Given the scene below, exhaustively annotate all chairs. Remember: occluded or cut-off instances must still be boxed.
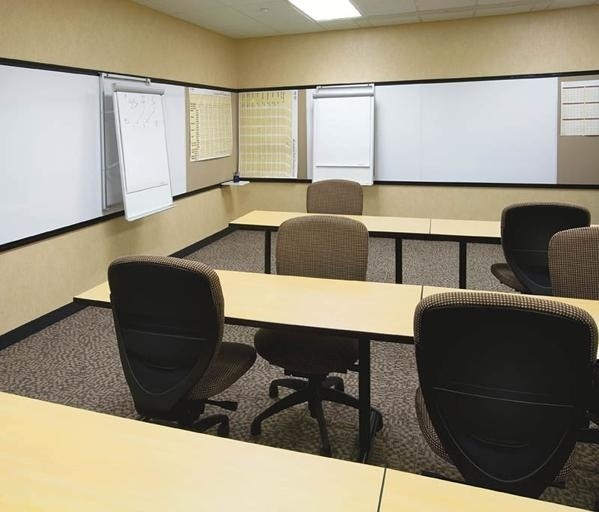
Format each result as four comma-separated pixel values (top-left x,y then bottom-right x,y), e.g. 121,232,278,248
105,181,599,500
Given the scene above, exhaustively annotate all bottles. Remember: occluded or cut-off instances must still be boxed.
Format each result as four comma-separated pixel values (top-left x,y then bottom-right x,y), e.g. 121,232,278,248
233,172,239,182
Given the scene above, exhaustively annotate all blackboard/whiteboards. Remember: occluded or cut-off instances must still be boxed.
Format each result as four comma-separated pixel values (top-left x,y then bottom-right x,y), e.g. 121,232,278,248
312,90,374,187
111,83,175,222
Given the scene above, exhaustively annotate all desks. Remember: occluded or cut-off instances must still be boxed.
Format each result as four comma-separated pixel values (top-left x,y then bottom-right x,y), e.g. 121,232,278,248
71,270,599,463
0,389,587,511
